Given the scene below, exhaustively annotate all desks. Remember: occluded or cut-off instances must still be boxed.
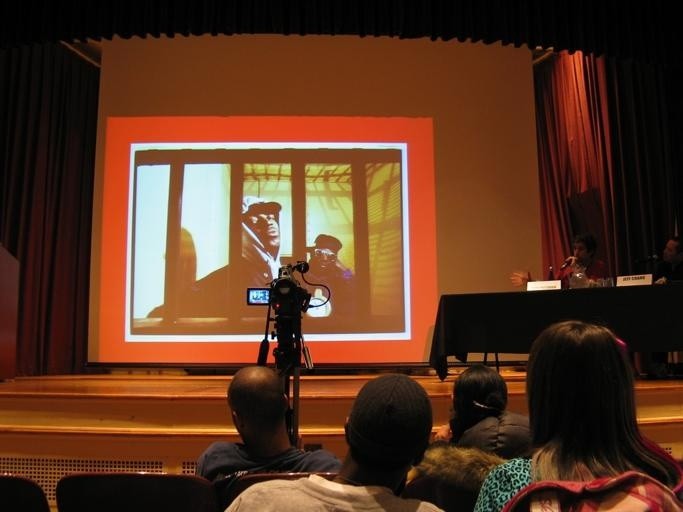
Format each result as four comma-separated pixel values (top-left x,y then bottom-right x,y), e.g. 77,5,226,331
429,281,683,381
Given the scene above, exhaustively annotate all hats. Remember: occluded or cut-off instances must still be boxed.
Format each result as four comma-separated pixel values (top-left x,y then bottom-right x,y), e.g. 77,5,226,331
314,233,343,254
344,371,434,463
241,195,282,219
501,470,683,512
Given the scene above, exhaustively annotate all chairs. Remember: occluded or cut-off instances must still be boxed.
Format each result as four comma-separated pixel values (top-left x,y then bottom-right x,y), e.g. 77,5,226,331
54,473,213,511
2,472,50,511
219,471,345,511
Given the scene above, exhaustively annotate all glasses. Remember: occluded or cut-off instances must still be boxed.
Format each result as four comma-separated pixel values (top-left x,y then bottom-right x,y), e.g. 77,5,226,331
314,248,336,263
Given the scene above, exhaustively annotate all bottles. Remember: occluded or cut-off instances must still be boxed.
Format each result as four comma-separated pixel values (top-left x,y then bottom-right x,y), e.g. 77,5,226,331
548,267,554,280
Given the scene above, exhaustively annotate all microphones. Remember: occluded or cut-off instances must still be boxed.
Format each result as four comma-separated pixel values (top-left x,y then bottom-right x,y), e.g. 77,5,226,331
635,255,658,264
560,258,571,270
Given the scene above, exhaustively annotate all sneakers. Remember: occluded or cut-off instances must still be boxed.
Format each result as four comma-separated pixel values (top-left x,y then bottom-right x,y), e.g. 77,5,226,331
647,363,673,378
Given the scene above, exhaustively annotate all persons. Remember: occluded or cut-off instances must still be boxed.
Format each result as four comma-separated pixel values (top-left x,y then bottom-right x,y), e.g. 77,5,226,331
401,440,506,512
145,194,282,322
642,235,682,380
431,360,536,460
220,374,448,512
472,318,683,512
193,364,342,483
507,232,619,290
305,233,354,317
